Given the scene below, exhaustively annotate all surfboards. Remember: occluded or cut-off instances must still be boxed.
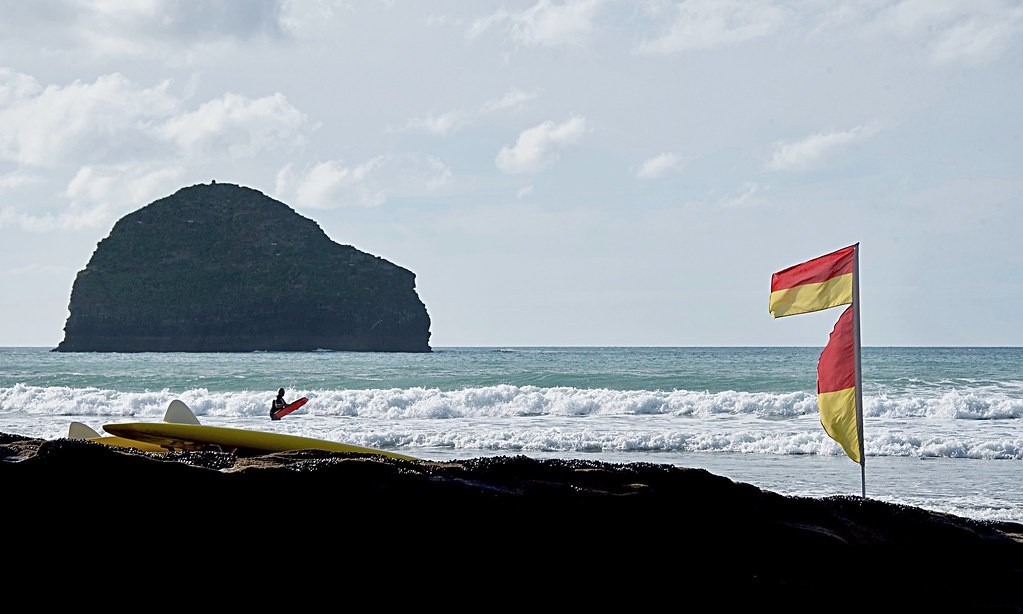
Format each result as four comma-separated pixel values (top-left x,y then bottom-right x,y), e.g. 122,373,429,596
275,397,308,418
67,400,418,461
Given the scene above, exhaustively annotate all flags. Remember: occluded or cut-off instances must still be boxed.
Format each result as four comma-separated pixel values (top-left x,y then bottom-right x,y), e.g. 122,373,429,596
769,244,865,467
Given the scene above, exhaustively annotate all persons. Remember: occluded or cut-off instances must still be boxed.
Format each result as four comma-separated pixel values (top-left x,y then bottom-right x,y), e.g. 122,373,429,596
269,387,289,420
